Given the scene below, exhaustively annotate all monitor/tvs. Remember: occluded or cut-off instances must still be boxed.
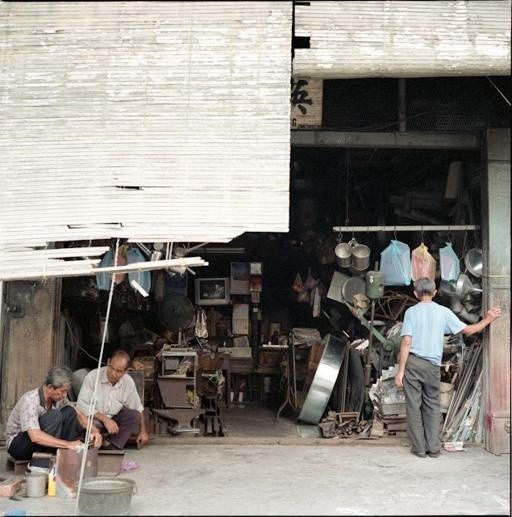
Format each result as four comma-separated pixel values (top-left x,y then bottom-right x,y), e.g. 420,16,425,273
195,277,229,306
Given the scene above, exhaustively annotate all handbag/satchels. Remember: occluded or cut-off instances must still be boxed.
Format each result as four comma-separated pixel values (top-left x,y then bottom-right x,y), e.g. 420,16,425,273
379,239,461,286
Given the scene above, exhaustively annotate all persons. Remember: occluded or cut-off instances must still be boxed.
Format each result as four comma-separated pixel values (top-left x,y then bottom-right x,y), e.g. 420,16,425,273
394,277,501,457
6,367,103,461
77,350,149,450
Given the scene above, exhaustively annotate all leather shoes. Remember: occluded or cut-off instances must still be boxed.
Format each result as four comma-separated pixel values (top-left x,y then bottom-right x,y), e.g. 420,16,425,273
411,448,440,458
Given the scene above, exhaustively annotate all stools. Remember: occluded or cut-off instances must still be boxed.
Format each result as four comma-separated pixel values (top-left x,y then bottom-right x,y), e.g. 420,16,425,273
6,455,30,474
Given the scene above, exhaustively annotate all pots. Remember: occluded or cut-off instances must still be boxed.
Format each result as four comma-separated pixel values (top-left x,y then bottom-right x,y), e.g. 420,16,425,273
70,477,137,515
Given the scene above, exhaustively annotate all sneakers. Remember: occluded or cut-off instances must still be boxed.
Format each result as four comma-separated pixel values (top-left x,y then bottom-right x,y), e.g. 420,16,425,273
99,442,118,450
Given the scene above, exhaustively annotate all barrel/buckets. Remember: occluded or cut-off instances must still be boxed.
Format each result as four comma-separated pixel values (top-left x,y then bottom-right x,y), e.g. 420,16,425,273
75,477,138,517
353,245,370,270
335,243,352,268
